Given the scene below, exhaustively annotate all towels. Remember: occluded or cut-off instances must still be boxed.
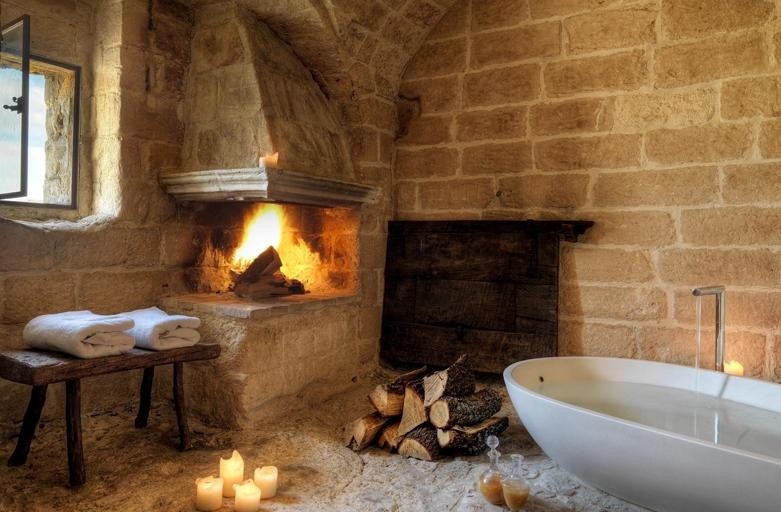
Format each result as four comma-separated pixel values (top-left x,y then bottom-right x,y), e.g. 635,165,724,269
22,305,202,359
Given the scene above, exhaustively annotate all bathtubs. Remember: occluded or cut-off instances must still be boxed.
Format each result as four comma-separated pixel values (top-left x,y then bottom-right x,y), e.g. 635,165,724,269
502,355,781,512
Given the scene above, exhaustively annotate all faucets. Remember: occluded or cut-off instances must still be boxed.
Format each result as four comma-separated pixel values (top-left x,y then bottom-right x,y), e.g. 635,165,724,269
691,285,725,370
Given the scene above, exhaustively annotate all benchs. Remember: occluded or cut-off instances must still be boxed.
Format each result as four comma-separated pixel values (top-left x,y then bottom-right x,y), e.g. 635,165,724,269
2,343,222,486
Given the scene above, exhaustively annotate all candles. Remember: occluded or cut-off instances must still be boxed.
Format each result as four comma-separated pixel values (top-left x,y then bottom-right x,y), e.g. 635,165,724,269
193,449,277,512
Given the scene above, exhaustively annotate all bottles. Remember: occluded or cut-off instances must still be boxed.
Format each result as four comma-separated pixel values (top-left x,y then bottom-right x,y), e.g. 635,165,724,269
478,434,509,506
498,452,533,512
